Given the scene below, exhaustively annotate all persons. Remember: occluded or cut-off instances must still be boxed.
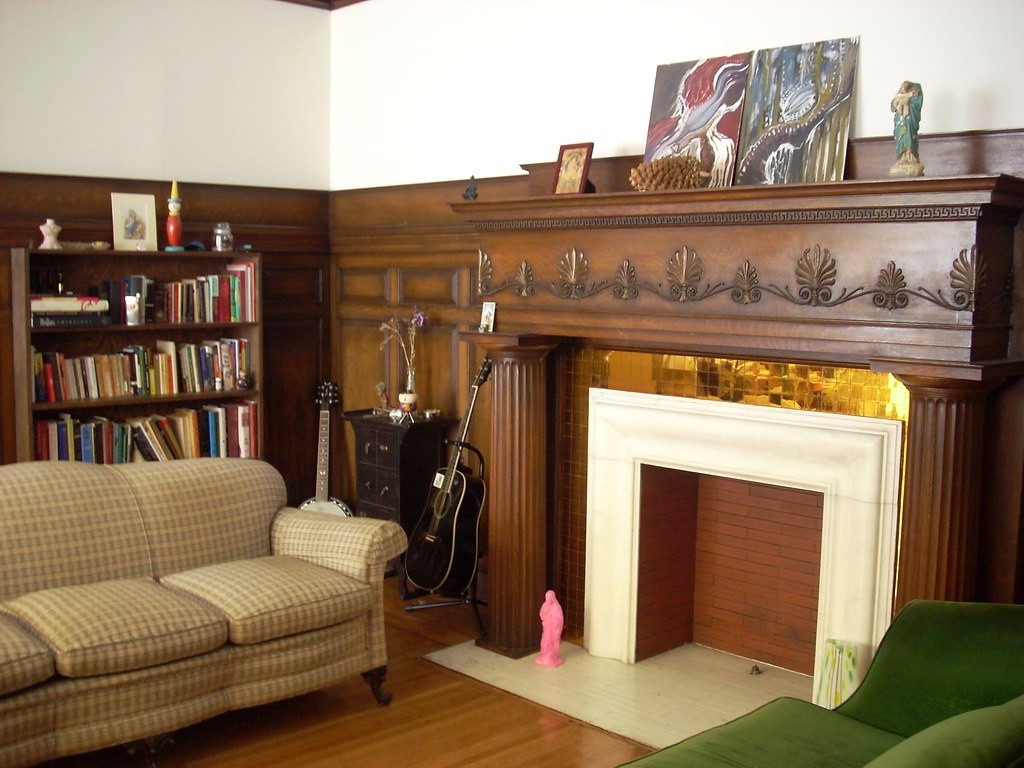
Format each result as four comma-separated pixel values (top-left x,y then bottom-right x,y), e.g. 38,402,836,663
535,590,565,668
462,175,478,200
373,381,392,416
39,219,63,249
891,81,924,163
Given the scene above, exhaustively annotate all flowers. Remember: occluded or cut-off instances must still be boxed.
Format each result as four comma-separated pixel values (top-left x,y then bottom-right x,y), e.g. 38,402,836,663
377,304,432,394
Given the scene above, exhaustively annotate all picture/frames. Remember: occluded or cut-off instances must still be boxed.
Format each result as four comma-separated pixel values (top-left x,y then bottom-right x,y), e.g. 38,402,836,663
480,301,496,333
111,192,158,252
551,142,594,194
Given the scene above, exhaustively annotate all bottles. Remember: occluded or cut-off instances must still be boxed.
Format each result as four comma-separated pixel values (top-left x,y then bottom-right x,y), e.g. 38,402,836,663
211,222,234,252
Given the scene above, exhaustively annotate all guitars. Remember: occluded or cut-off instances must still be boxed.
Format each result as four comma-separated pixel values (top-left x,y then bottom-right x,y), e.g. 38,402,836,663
402,352,492,598
296,379,353,517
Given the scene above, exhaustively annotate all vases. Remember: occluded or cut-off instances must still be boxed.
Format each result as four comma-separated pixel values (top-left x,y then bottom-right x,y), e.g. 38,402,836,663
398,367,421,413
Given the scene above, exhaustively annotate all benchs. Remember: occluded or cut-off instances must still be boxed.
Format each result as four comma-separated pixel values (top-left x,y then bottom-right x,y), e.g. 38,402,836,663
0,457,412,768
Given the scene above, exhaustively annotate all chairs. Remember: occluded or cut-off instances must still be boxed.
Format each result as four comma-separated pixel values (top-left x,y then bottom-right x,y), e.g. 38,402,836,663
611,598,1024,768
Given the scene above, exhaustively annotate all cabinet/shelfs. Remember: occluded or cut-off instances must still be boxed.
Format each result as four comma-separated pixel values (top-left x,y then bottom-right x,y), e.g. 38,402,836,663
10,246,267,465
339,407,461,571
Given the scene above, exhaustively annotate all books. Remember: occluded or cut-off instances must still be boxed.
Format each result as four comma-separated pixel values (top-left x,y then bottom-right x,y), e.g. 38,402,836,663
29,261,258,464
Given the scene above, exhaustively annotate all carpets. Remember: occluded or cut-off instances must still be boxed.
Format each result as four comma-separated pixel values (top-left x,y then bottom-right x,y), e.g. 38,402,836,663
422,636,815,751
